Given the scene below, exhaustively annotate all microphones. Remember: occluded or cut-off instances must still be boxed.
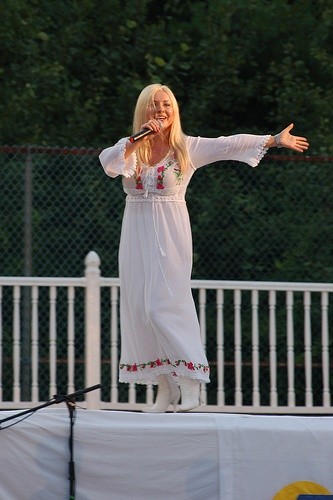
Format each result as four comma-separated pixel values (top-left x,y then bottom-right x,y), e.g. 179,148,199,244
130,120,163,143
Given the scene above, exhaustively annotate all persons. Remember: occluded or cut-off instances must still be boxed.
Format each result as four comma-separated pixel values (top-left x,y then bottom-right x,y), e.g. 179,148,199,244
98,83,309,412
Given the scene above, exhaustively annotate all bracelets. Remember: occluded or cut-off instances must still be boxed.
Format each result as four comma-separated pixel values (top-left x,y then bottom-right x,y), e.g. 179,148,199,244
274,134,282,149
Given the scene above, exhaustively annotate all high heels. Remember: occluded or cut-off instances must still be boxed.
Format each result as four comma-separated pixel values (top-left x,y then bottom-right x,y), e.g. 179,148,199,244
177,382,204,411
143,385,181,413
